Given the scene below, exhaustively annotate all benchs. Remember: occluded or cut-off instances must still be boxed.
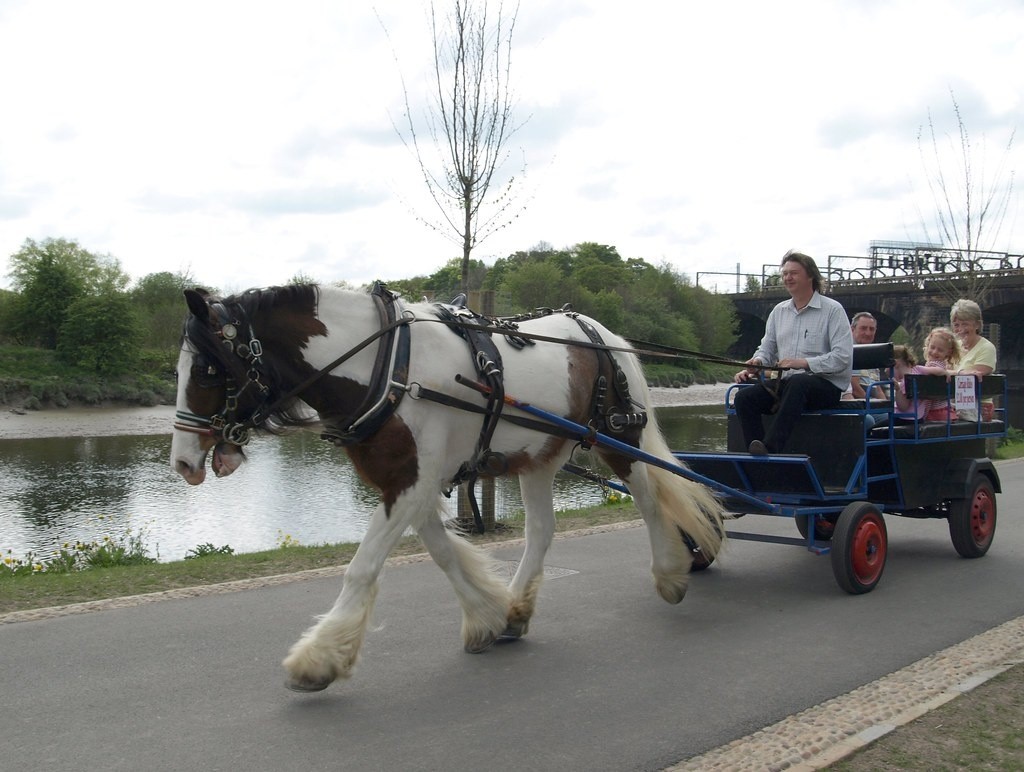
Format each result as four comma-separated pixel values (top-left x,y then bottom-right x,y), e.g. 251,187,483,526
726,341,1010,487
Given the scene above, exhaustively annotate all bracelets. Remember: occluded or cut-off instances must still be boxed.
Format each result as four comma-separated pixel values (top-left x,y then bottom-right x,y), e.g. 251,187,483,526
957,368,965,376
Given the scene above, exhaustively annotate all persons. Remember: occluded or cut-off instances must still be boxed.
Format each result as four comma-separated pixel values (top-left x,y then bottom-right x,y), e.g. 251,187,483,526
923,328,961,421
867,343,951,438
735,360,874,431
851,312,889,430
946,299,996,422
735,253,853,456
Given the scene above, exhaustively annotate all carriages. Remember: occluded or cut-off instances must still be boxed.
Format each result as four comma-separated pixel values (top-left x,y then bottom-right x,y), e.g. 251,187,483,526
169,278,1008,695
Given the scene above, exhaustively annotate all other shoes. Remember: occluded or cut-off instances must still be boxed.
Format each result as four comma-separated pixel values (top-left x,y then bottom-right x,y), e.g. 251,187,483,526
748,440,769,455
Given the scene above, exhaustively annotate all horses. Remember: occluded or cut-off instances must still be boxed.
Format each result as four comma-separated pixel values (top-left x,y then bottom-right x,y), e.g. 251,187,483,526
168,281,736,693
